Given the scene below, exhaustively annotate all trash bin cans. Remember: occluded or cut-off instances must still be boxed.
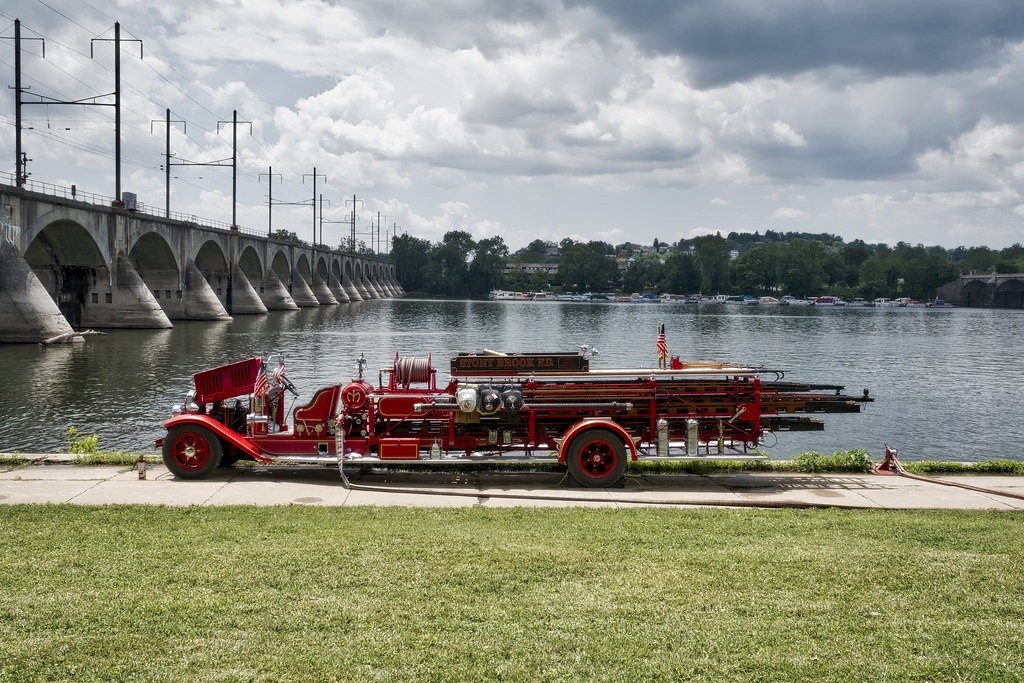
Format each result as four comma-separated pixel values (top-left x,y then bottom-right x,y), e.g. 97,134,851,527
122,192,137,210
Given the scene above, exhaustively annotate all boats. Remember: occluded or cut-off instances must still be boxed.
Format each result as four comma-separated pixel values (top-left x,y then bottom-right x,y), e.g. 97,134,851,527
488,289,954,308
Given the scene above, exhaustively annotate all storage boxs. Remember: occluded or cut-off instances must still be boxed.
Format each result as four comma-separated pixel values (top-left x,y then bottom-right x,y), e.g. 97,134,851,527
378,437,421,460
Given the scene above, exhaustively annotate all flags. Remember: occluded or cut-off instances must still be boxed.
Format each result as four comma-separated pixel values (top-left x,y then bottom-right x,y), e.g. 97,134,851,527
272,357,282,385
254,357,269,400
275,356,286,390
656,323,668,360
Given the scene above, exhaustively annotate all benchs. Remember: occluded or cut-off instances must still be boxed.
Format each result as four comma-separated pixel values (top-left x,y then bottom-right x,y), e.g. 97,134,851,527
292,382,343,438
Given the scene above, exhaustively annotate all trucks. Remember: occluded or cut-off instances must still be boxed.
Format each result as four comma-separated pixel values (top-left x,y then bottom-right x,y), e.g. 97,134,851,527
150,347,762,488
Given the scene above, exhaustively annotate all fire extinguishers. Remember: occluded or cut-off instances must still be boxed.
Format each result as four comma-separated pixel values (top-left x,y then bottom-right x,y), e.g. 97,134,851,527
131,454,147,480
